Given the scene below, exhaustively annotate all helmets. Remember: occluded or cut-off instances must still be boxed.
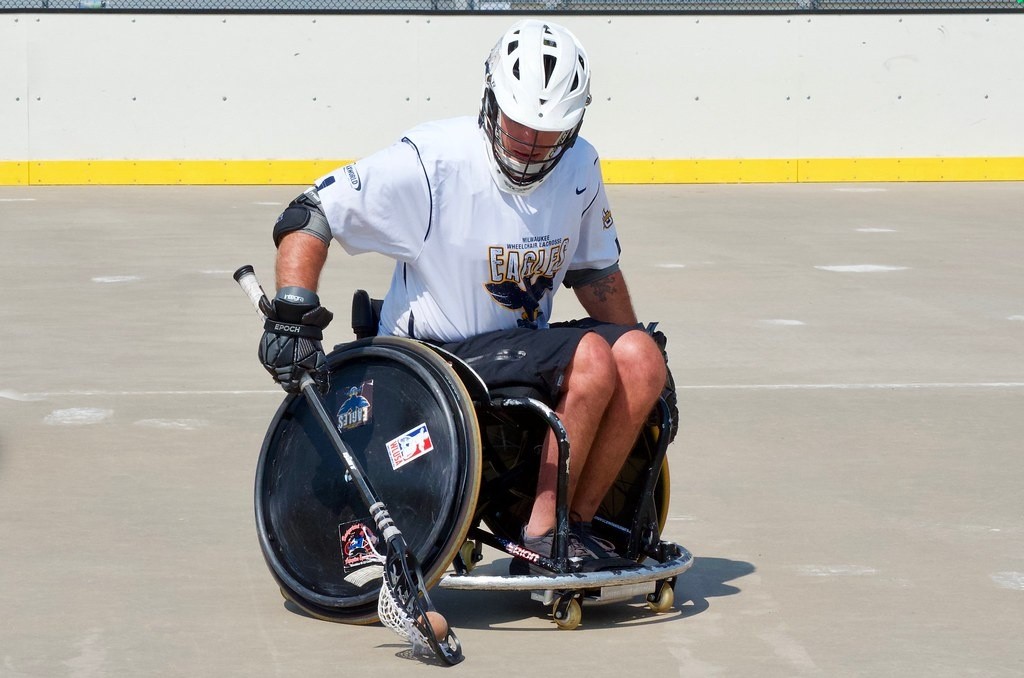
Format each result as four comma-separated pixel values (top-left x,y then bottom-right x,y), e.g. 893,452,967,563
477,19,592,196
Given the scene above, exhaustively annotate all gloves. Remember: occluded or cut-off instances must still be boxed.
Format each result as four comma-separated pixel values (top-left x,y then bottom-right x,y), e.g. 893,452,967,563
256,297,330,398
636,321,680,445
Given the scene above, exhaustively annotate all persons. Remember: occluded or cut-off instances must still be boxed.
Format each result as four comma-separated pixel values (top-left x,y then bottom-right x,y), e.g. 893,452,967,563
257,19,668,573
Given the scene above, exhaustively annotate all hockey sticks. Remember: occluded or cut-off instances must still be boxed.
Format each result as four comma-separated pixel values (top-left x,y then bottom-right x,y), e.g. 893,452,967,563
233,262,463,666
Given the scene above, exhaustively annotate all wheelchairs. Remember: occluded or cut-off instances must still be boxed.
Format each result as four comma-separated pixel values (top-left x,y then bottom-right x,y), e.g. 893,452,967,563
252,290,695,630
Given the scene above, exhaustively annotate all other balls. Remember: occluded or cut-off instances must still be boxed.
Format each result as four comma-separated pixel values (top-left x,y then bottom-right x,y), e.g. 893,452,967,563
415,610,450,642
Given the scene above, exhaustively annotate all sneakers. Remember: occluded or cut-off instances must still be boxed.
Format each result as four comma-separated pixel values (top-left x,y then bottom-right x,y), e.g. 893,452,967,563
522,530,620,566
